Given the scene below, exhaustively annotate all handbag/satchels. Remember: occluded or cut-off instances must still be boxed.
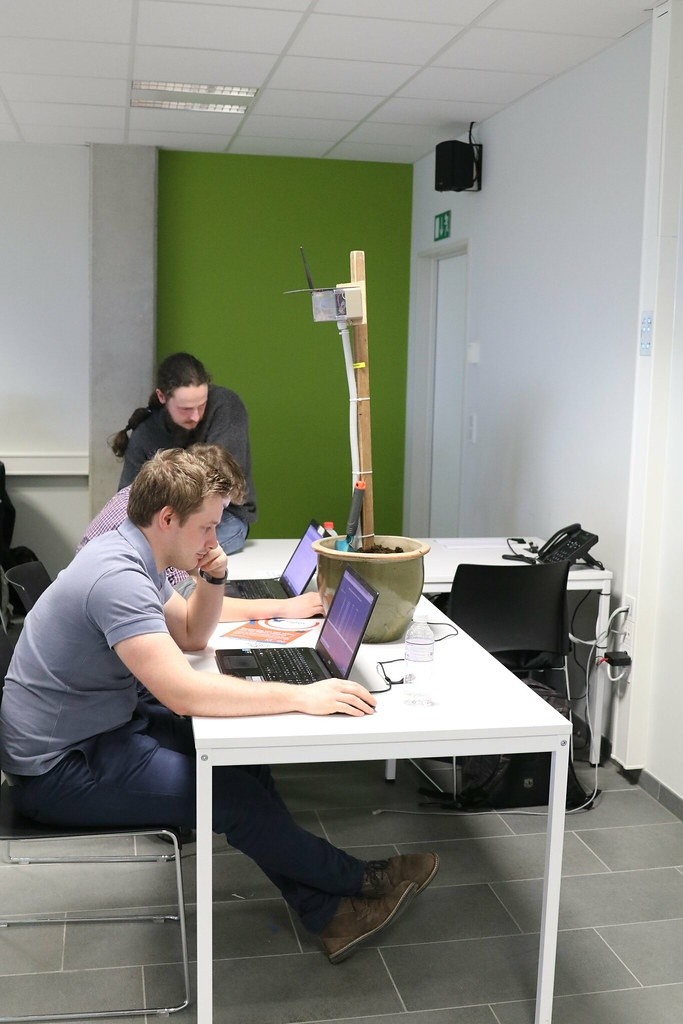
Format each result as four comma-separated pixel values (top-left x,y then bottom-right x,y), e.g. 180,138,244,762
420,752,602,810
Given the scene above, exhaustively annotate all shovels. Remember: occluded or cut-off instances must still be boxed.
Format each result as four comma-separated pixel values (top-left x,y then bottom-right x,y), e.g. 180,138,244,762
335,477,366,553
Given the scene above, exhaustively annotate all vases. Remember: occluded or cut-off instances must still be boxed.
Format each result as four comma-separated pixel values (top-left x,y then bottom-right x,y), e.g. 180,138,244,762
312,535,431,643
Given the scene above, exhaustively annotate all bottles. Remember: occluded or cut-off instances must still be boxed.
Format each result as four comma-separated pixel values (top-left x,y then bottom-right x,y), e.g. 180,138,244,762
323,521,338,536
405,611,435,708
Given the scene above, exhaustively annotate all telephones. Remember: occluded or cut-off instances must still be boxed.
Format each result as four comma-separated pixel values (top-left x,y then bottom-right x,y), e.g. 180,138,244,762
537,523,599,565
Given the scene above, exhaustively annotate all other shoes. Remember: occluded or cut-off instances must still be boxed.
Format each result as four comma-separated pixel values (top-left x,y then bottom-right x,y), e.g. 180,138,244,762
322,880,419,965
362,851,439,894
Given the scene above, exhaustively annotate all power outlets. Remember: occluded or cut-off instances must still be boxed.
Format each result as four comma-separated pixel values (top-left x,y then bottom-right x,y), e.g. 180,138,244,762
620,619,635,649
618,643,634,683
623,594,637,622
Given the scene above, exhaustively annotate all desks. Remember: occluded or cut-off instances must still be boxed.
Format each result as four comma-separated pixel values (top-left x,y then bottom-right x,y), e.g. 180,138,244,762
184,594,573,1024
226,537,613,779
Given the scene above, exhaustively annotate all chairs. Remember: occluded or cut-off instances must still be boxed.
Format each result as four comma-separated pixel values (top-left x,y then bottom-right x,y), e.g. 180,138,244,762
0,559,193,1024
446,559,574,800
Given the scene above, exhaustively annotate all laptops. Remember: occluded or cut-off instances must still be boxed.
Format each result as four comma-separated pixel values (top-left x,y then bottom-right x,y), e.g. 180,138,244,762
215,564,380,686
224,518,332,600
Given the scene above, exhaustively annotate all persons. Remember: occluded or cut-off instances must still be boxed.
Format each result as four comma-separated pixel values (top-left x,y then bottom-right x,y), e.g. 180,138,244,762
0,445,440,964
115,352,257,556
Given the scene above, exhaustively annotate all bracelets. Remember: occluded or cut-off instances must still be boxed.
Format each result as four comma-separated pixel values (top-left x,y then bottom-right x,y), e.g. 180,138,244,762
198,568,228,585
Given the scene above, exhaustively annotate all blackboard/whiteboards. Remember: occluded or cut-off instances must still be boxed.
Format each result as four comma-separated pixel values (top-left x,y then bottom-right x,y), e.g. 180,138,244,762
0,141,92,476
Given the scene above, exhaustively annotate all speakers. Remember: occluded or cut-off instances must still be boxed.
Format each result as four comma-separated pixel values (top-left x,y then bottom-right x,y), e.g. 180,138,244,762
434,140,474,192
